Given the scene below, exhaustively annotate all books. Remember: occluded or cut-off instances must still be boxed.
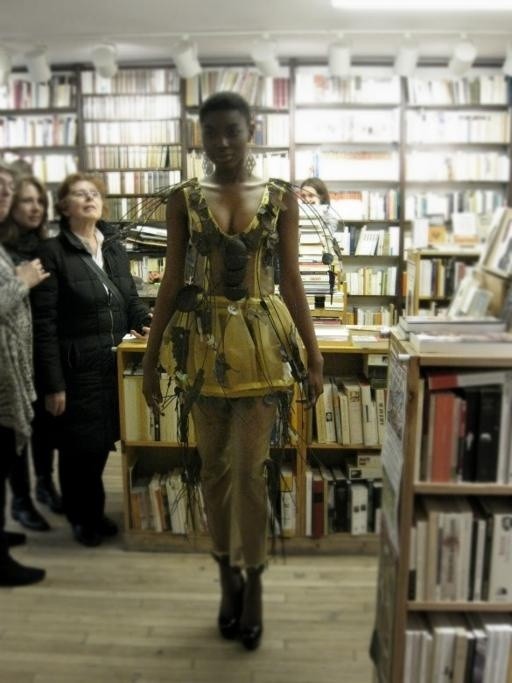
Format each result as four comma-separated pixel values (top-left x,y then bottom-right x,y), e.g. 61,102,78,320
81,68,182,93
126,225,170,247
419,302,451,316
83,95,181,119
409,220,487,247
406,148,510,181
43,224,60,240
409,76,511,104
306,455,382,538
405,187,509,220
409,497,512,603
392,311,512,357
354,334,380,342
103,197,168,220
294,110,401,145
347,304,404,328
84,122,182,144
128,258,167,291
330,225,401,256
406,110,512,144
270,465,297,538
295,74,403,105
1,78,77,108
97,172,181,193
330,189,400,220
0,116,78,147
124,366,196,445
188,149,214,183
85,147,184,170
415,369,512,486
271,381,301,446
43,187,63,220
184,112,205,148
129,463,208,536
186,70,291,109
307,354,391,445
314,325,349,340
404,610,512,682
248,153,291,187
342,266,399,297
449,208,512,310
297,199,346,312
3,154,78,183
296,148,403,182
412,259,471,297
251,112,292,148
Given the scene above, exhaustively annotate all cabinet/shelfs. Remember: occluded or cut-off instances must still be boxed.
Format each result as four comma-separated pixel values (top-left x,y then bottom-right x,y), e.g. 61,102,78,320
116,336,390,555
78,69,185,286
371,332,512,683
0,68,80,241
400,66,512,318
291,63,403,331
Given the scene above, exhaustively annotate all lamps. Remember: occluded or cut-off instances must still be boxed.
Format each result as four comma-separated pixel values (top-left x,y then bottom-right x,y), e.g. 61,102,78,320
394,32,422,79
169,32,203,78
251,33,281,75
448,33,478,75
182,66,292,186
21,46,52,84
328,37,354,74
89,35,120,79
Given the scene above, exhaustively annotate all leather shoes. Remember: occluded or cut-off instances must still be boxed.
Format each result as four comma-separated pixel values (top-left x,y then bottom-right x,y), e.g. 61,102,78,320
1,561,46,585
92,513,120,536
5,529,28,546
74,522,104,546
38,486,64,510
10,498,50,533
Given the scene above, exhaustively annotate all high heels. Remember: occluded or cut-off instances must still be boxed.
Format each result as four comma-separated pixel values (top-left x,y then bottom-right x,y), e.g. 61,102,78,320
240,598,264,647
217,572,245,636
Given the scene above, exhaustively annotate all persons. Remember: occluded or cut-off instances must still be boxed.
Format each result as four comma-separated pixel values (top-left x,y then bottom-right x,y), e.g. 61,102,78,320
38,173,151,547
141,96,324,652
301,179,327,205
0,173,50,268
1,167,52,590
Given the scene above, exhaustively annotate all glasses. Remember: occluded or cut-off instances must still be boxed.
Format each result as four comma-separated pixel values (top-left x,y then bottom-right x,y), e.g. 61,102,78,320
0,179,17,192
65,189,104,199
